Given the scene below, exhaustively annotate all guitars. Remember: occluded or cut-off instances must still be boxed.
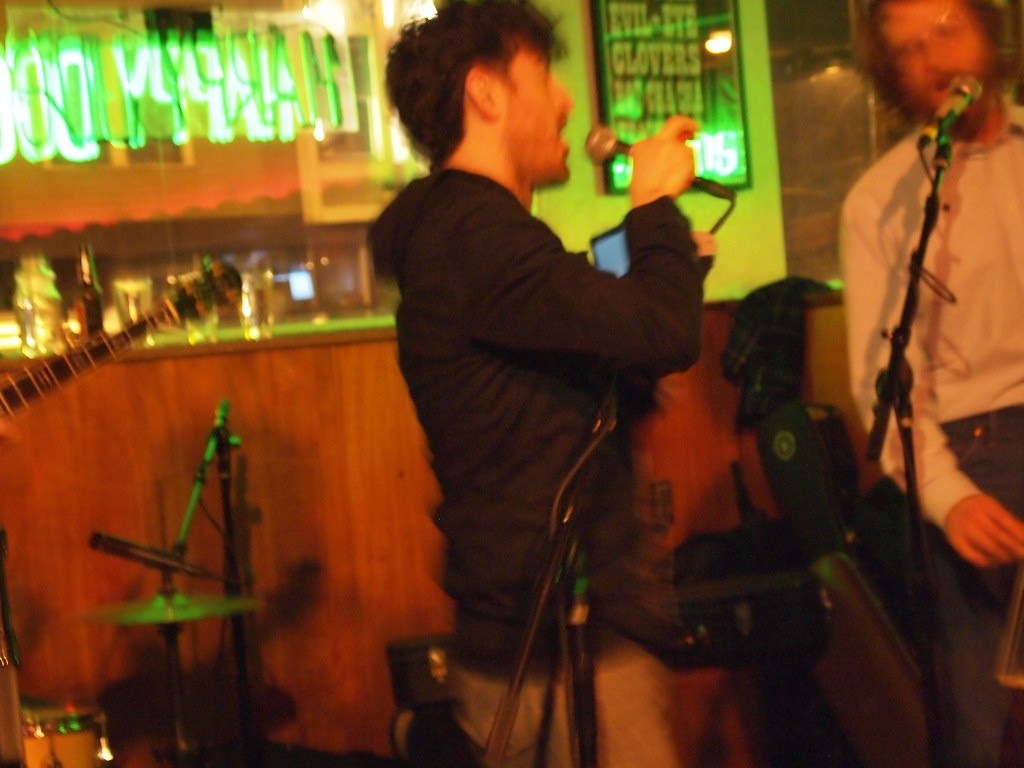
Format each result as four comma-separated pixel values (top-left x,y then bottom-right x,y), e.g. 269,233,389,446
2,259,245,421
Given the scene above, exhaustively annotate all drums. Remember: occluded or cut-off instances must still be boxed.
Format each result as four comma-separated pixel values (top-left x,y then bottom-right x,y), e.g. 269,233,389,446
18,698,115,768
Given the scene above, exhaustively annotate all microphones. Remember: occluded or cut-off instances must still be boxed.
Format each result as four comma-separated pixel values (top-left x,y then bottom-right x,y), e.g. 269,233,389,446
585,125,734,201
89,530,209,578
918,75,982,151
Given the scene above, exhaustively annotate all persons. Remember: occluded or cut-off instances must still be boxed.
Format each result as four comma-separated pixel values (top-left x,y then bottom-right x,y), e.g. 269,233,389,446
837,0,1024,767
362,3,718,768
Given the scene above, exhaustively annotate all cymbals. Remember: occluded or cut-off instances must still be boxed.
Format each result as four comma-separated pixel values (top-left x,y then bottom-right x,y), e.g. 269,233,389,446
78,587,260,626
